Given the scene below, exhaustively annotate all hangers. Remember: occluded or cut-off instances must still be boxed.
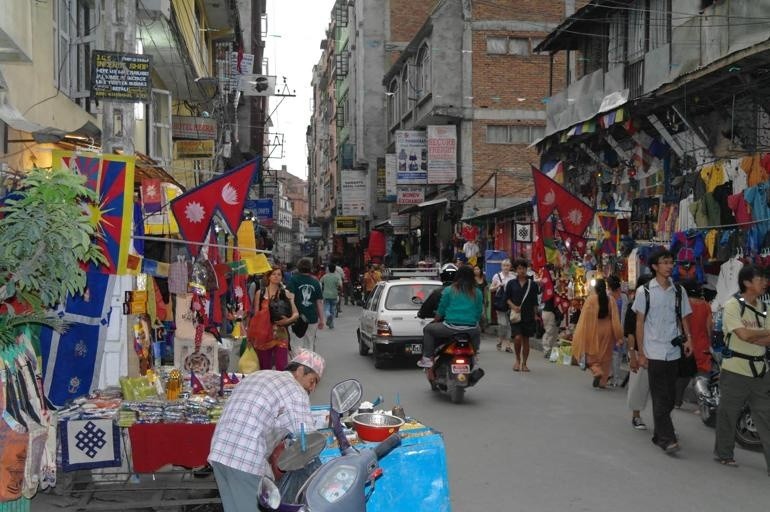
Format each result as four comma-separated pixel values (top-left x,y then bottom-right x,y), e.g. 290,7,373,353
735,247,769,260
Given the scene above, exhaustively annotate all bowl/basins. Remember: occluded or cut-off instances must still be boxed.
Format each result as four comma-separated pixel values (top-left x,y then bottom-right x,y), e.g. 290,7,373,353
351,413,406,443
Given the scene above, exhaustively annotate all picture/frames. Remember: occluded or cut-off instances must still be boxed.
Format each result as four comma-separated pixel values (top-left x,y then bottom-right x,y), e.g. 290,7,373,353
174,339,219,380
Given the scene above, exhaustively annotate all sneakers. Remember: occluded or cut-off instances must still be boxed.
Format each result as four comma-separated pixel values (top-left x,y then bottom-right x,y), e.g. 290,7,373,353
592,374,602,387
631,415,647,431
496,343,502,351
505,346,513,353
544,349,551,358
326,315,333,328
416,357,434,368
652,436,681,453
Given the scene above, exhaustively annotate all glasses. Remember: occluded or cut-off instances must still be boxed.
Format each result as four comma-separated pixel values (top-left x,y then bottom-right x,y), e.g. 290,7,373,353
658,262,674,264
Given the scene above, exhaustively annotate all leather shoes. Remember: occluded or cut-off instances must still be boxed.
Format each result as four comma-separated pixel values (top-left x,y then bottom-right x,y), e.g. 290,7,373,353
513,367,520,371
522,366,530,371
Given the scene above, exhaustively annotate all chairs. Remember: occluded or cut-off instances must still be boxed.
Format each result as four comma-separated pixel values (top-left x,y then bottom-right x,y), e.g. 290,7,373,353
390,292,410,305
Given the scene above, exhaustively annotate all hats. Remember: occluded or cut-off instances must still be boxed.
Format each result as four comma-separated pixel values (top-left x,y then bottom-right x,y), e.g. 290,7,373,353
296,257,312,269
288,350,326,381
439,263,458,275
455,252,467,263
418,261,425,265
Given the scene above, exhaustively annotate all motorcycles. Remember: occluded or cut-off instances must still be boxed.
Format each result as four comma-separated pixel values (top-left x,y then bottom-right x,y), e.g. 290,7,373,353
254,379,403,512
411,297,484,404
693,329,764,451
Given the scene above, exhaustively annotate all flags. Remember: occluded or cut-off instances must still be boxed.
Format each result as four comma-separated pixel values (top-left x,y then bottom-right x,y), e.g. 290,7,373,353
51,148,135,278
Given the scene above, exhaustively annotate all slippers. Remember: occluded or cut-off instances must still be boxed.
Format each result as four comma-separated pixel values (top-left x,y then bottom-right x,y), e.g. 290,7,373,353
715,457,739,466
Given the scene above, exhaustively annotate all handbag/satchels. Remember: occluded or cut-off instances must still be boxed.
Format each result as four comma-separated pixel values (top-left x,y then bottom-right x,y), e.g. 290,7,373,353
491,272,510,313
290,317,308,338
678,349,698,379
269,284,293,328
510,306,522,324
535,319,546,339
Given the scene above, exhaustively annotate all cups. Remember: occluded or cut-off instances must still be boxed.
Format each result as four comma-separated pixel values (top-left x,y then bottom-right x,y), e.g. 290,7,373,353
392,407,405,419
359,408,373,414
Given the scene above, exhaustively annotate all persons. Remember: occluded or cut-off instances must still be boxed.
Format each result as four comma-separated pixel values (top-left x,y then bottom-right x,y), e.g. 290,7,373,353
629,249,694,459
417,254,488,368
572,274,715,428
489,257,540,372
360,260,390,306
715,265,770,478
204,348,328,512
253,255,354,370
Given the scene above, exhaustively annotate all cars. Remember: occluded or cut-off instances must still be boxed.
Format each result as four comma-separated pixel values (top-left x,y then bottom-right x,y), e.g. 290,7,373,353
357,266,444,371
